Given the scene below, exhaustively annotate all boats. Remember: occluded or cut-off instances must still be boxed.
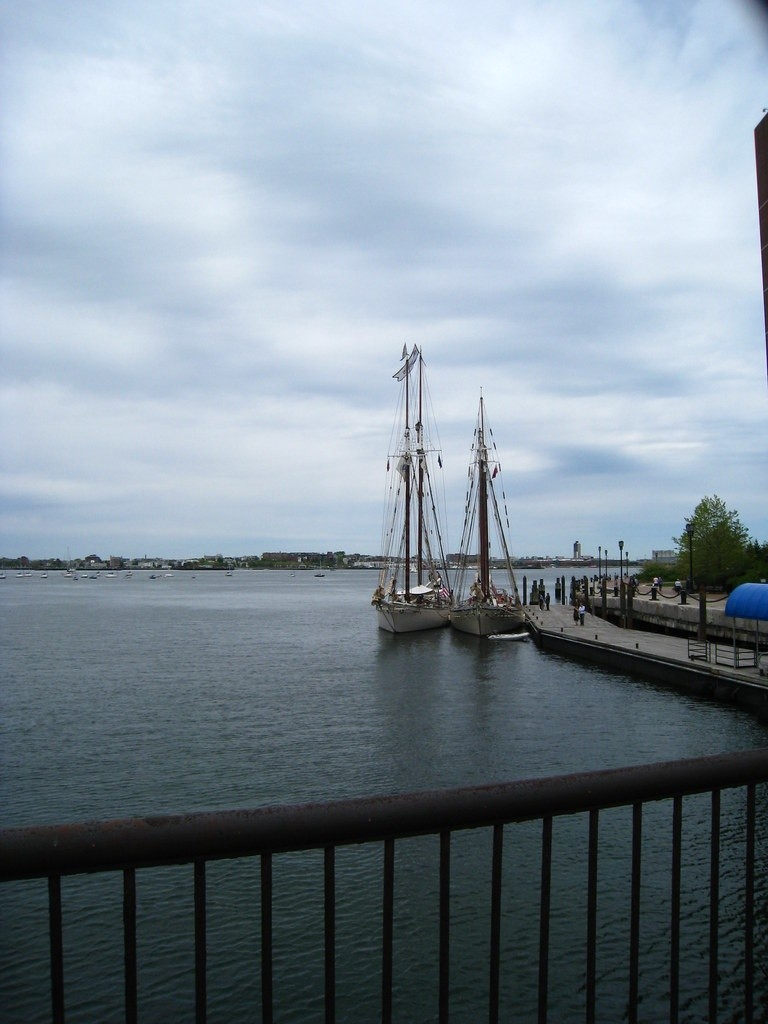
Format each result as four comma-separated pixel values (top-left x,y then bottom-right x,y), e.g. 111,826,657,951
1,568,235,580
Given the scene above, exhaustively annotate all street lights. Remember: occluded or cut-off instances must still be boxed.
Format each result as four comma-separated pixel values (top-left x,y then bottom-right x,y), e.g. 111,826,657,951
598,546,602,584
626,551,630,577
618,540,625,584
685,523,697,594
604,549,608,577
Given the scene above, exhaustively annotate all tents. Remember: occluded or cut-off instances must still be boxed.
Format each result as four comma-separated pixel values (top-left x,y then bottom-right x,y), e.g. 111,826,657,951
725,582,768,669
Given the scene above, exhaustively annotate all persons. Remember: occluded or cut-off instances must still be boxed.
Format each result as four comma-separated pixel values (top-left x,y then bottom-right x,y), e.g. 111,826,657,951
539,592,550,610
573,603,586,626
653,576,663,591
675,578,681,595
634,577,640,592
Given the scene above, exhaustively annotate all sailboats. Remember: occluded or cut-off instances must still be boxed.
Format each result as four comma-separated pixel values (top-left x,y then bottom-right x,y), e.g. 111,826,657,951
368,342,455,636
449,386,528,639
